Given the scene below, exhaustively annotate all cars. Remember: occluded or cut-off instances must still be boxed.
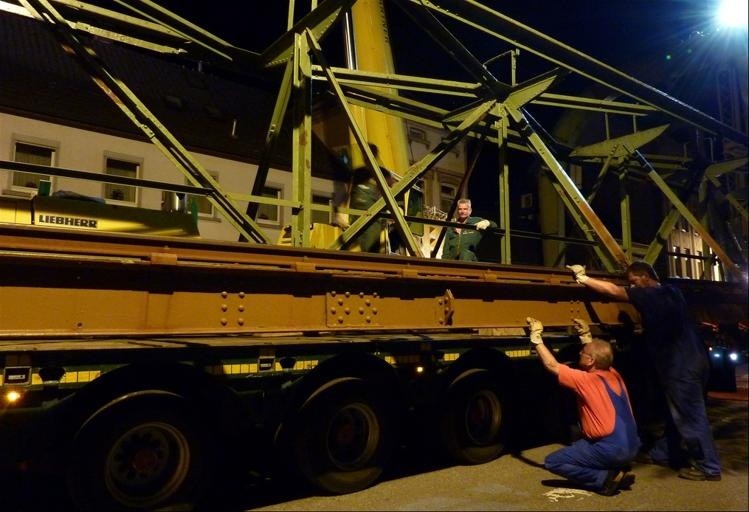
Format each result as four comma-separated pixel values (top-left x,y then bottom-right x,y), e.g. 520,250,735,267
712,347,739,365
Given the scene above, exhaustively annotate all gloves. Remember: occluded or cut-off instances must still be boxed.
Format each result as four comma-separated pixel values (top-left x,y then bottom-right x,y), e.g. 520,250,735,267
526,317,543,345
573,318,592,344
474,219,490,231
566,264,588,284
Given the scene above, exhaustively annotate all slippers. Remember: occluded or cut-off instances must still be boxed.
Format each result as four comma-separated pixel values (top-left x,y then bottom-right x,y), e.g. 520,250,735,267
602,471,636,495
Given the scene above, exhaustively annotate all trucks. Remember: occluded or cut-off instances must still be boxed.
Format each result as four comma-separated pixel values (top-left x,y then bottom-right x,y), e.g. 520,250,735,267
2,190,748,512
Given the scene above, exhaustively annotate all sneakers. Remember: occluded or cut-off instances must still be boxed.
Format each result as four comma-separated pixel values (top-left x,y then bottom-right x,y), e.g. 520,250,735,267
637,450,653,464
680,466,721,481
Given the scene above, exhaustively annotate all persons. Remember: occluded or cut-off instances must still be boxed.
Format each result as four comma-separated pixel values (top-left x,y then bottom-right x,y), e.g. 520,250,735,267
525,313,642,497
566,256,722,484
353,143,408,255
446,198,498,263
